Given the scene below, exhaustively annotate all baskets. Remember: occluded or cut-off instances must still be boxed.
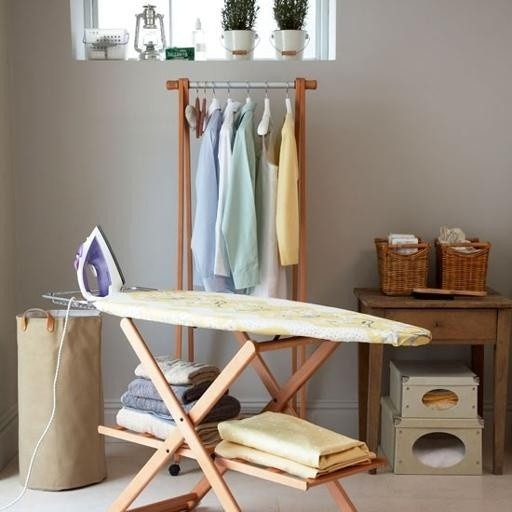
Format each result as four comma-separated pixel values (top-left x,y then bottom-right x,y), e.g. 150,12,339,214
375,236,432,298
435,235,491,292
82,27,129,59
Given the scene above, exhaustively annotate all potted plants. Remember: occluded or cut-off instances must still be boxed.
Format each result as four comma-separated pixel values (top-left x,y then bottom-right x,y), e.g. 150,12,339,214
268,1,311,60
220,0,263,60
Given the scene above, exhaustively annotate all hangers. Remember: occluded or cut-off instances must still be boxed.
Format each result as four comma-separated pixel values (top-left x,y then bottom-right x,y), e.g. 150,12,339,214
185,80,298,140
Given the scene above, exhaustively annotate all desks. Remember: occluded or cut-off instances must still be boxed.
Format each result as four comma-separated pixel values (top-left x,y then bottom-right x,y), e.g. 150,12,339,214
351,286,511,475
41,285,433,512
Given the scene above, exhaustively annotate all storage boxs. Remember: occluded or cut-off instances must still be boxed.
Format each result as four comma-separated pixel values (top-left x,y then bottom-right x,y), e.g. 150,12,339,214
389,360,480,417
377,395,484,476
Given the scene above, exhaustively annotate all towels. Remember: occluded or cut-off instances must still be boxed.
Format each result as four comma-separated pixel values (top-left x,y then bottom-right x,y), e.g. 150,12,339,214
215,410,370,467
212,437,369,479
115,409,223,447
125,378,230,404
120,390,241,423
132,356,221,385
